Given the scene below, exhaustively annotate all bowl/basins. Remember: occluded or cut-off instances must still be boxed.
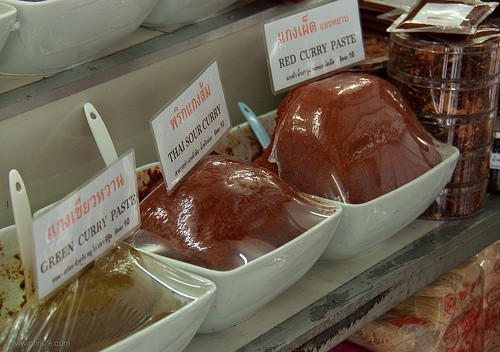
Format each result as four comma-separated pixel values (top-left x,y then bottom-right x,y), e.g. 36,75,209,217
207,108,459,260
124,162,342,335
0,222,213,352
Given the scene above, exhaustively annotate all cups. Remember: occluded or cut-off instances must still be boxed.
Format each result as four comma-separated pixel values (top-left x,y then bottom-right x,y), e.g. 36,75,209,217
335,35,388,77
386,30,499,219
